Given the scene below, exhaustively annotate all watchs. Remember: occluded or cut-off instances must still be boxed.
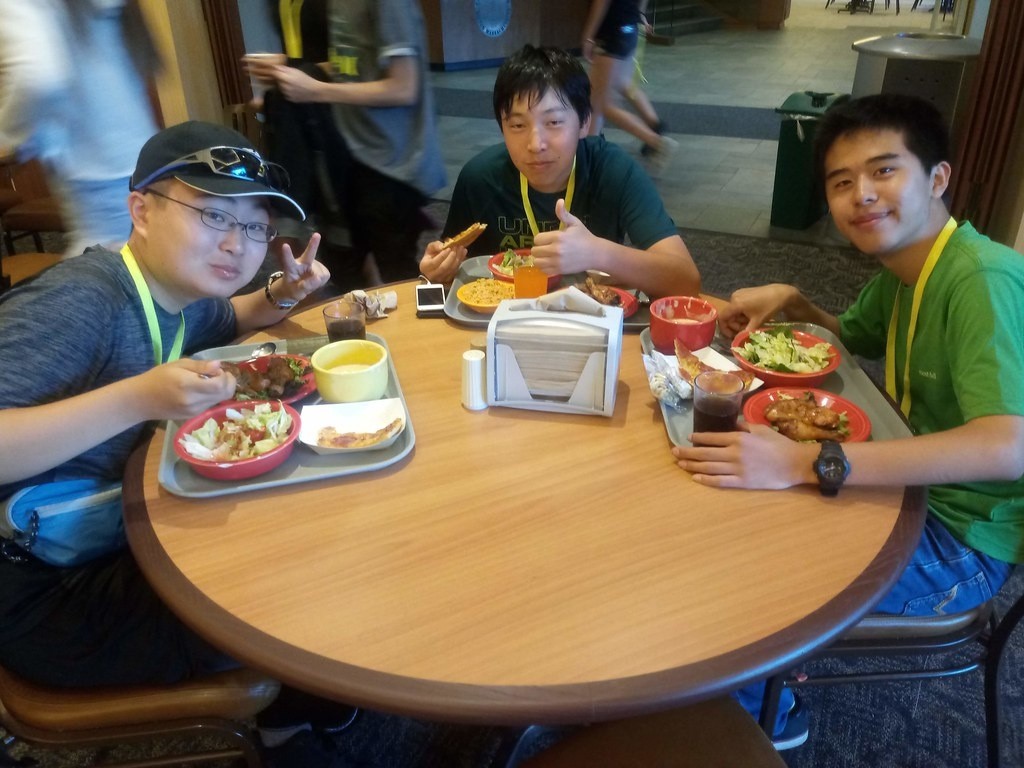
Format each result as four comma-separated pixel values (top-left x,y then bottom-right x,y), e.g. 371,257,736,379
265,271,299,309
813,441,851,497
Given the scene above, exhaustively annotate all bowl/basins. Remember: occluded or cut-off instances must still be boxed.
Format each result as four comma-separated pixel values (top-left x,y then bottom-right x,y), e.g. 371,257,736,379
649,297,717,353
488,249,562,289
311,340,388,402
731,328,841,391
173,399,301,480
457,278,514,313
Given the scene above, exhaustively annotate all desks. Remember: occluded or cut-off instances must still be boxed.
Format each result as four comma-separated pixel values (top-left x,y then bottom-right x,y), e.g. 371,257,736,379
122,279,929,728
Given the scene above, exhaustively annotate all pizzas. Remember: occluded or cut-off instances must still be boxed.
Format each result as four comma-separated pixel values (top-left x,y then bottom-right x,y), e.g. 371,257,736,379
443,223,487,248
674,337,755,389
318,418,401,447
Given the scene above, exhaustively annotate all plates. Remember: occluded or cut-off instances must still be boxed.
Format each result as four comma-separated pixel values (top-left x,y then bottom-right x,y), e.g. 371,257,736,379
215,355,316,406
744,385,871,448
558,285,639,319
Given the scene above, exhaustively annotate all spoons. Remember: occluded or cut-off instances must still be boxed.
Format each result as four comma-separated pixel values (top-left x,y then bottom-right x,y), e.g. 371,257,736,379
204,343,277,380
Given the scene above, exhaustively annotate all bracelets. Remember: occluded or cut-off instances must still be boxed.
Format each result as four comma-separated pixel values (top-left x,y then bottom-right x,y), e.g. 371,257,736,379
586,39,594,44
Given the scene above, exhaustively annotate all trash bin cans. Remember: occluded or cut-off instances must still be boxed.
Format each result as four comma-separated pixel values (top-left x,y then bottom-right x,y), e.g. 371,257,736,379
851,33,984,169
769,88,850,231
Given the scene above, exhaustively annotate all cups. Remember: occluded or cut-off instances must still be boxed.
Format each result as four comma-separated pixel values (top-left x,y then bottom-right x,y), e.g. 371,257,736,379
322,303,366,343
693,371,744,447
513,257,548,301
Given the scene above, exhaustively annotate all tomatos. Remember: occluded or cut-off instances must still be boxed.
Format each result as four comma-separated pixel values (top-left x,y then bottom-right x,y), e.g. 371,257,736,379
245,429,265,443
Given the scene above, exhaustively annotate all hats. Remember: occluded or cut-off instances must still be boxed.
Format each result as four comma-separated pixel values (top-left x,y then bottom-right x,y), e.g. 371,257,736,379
129,119,306,222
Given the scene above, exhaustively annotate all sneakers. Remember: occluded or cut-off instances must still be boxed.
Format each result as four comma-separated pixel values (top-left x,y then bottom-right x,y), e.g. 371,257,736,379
772,690,809,751
257,692,359,748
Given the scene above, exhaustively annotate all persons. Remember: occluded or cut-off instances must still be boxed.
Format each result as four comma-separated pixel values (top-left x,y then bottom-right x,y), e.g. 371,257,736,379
420,44,700,299
241,0,447,294
0,120,358,743
671,93,1024,751
0,0,162,254
580,0,680,178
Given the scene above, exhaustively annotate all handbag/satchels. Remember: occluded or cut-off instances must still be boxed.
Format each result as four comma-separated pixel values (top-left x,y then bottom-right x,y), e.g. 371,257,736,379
0,476,129,567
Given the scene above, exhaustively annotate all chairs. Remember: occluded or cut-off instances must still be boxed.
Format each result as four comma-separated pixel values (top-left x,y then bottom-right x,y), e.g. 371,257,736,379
760,564,1024,768
0,153,77,295
489,695,791,768
0,663,282,768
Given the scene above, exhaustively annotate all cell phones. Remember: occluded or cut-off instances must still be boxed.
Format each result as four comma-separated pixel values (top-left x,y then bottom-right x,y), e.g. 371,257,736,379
415,284,446,311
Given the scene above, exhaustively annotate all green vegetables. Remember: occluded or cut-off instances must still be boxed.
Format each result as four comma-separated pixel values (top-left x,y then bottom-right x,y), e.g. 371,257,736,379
771,390,850,445
235,357,313,401
729,323,836,372
493,248,535,276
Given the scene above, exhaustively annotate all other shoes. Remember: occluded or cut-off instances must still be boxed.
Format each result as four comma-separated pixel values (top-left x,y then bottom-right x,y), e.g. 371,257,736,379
648,135,681,179
640,120,671,157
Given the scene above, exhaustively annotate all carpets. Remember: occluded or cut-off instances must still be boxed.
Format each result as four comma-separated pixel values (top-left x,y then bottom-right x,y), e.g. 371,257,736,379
1,199,1024,768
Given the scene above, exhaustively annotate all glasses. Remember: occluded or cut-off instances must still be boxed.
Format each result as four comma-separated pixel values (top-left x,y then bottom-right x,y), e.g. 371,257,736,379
134,145,292,195
139,186,279,243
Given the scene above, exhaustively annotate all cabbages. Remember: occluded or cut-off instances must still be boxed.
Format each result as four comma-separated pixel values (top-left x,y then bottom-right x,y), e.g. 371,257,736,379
177,400,292,461
